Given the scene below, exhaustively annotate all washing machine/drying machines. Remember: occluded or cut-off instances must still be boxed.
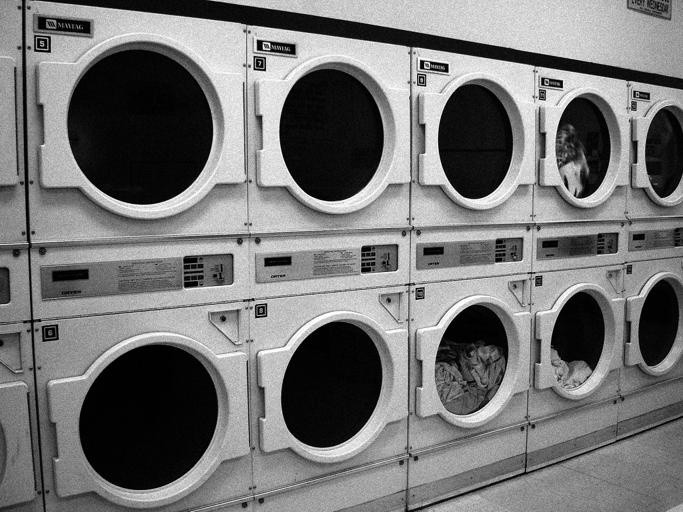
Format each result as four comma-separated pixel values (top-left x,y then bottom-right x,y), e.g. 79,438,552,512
1,2,683,511
